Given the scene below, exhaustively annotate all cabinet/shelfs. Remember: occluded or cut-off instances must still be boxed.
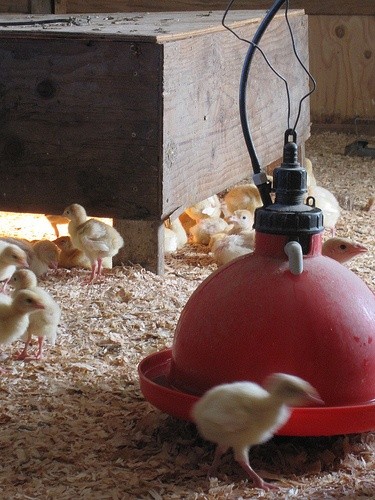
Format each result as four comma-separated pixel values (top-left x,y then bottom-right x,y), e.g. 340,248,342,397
1,6,312,277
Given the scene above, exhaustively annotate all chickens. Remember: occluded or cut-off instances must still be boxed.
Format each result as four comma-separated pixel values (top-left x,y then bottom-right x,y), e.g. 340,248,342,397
5,268,59,360
61,203,124,285
0,235,113,285
189,370,327,493
321,236,370,264
178,175,276,266
300,157,342,238
0,289,48,357
